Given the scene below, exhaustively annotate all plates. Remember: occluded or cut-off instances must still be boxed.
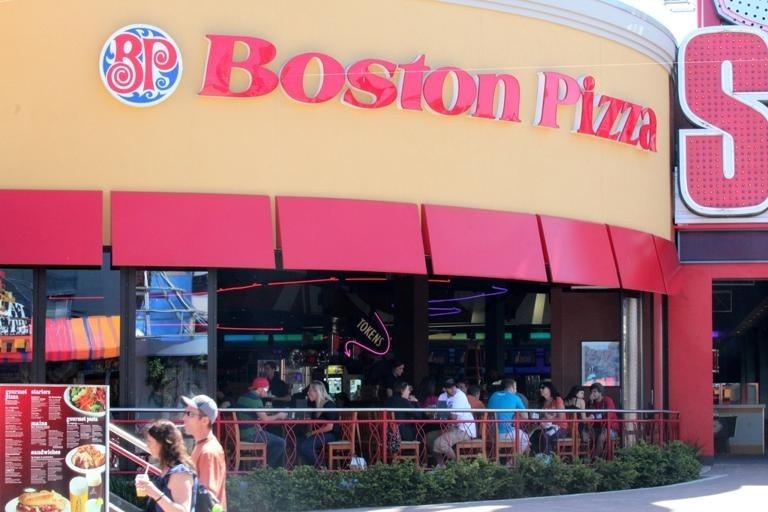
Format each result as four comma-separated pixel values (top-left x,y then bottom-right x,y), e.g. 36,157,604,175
65,443,106,474
4,496,71,512
63,387,106,418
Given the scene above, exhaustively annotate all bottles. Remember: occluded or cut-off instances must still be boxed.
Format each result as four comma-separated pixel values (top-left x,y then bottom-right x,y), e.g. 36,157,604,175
264,401,274,417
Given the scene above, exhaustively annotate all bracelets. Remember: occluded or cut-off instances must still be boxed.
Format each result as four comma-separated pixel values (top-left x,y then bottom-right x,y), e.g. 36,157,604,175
154,492,165,504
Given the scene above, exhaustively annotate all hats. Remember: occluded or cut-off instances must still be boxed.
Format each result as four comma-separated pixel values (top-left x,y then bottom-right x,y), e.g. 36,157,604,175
440,376,459,387
247,377,269,390
181,394,219,425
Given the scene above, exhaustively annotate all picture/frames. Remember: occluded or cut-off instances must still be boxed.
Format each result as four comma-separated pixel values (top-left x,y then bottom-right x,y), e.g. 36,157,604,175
580,340,621,390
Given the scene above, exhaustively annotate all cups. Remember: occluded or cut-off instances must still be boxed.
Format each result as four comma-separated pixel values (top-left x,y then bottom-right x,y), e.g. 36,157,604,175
69,472,102,512
135,473,149,497
288,411,296,419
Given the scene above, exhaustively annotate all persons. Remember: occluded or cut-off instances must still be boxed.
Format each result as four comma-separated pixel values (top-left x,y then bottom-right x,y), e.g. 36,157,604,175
487,377,618,464
262,362,292,406
222,376,341,471
378,361,486,469
134,418,197,511
181,395,228,512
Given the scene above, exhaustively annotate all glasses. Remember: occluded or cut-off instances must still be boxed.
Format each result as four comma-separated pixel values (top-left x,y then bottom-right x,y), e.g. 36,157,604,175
182,410,206,418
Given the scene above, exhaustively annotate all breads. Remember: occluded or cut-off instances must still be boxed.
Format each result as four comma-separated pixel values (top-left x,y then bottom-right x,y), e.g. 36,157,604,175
18,490,54,506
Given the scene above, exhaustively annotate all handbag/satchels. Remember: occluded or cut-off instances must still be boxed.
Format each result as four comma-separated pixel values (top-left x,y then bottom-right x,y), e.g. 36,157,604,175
195,485,220,511
349,454,368,472
